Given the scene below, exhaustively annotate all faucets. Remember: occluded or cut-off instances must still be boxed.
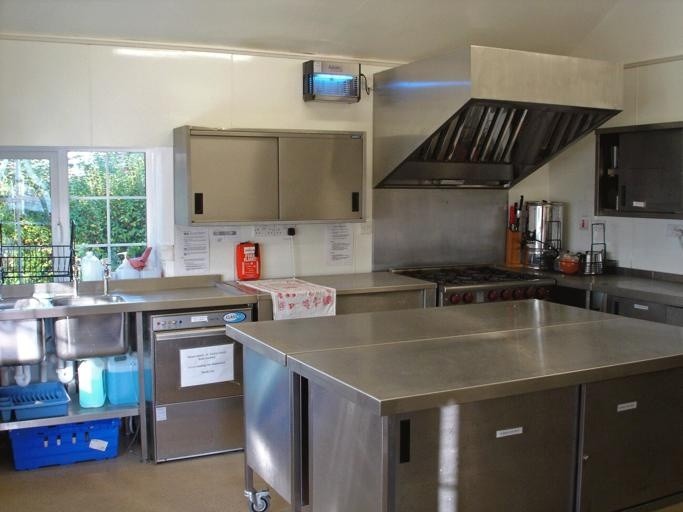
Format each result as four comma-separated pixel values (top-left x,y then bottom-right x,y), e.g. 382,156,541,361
102,262,111,295
71,265,81,299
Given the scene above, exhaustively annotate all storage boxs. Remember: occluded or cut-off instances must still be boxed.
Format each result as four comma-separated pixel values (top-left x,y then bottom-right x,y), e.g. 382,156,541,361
8,416,123,470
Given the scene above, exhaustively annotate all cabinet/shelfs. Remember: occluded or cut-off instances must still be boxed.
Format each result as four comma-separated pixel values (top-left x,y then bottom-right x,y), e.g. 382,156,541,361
611,296,683,328
172,124,366,227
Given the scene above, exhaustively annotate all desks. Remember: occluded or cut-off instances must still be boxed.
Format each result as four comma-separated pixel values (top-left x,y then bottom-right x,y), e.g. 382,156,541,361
286,316,683,512
224,297,619,512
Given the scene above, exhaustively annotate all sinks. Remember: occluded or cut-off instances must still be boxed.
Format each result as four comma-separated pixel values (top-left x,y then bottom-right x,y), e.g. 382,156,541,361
0,298,40,313
48,295,129,309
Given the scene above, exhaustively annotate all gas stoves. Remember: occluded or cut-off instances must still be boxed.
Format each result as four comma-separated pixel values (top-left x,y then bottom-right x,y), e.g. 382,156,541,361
391,265,553,283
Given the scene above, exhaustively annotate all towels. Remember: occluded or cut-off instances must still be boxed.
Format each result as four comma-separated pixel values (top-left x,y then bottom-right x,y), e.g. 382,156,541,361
236,278,338,321
